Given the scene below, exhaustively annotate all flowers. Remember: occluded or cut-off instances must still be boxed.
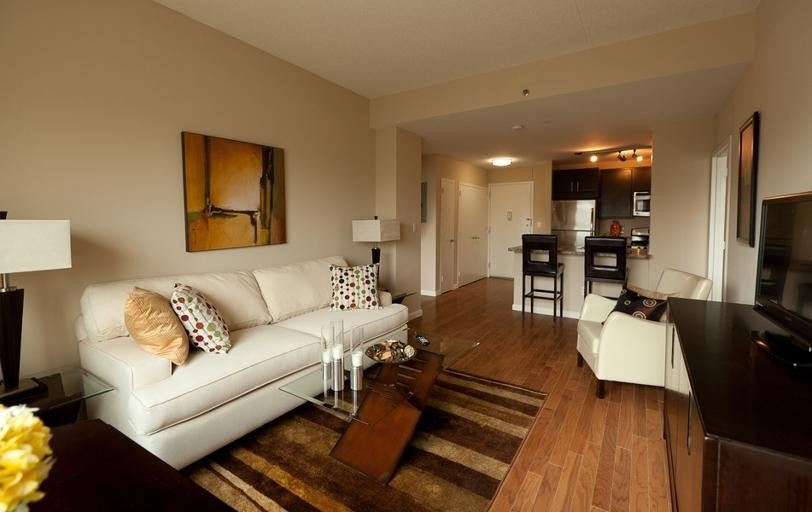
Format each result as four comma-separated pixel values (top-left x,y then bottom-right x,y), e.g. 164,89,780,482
0,403,56,512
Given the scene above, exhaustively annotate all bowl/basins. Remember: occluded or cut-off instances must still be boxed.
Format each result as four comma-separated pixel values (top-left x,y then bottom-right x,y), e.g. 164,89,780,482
366,340,417,366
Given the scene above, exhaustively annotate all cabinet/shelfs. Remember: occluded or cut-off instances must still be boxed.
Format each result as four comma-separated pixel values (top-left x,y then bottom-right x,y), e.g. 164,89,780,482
29,418,243,512
632,167,651,194
551,168,598,200
662,296,812,512
597,167,632,219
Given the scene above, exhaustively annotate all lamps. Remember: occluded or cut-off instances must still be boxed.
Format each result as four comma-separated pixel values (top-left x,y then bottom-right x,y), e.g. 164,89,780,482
617,152,627,162
0,211,76,404
351,215,401,293
632,150,643,162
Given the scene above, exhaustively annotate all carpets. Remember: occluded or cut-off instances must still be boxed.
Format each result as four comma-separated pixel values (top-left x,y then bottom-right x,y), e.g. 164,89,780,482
179,362,550,512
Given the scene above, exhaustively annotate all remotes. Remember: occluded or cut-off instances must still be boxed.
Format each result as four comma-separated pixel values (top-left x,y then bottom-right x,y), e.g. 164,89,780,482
415,335,430,346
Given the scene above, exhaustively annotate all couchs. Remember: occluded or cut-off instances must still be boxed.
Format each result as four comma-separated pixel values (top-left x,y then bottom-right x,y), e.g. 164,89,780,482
78,255,410,471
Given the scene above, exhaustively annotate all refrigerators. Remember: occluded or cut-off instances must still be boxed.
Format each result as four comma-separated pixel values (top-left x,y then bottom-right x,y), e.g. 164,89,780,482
553,199,600,253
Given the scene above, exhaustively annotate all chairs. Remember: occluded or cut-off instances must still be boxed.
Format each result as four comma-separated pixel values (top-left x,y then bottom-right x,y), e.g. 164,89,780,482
575,267,712,398
584,236,628,301
520,234,565,322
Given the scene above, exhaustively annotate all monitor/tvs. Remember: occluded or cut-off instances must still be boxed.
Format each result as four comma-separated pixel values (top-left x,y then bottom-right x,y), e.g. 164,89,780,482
751,191,812,368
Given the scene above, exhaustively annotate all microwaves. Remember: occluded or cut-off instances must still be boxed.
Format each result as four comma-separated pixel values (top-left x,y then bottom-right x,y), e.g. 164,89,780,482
633,192,651,217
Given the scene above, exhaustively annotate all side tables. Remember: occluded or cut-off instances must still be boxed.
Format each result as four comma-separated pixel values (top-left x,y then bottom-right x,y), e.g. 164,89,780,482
0,365,115,427
386,288,421,305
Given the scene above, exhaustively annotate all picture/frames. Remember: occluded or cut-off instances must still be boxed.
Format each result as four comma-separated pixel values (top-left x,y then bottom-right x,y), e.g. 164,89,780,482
735,112,758,248
421,180,429,225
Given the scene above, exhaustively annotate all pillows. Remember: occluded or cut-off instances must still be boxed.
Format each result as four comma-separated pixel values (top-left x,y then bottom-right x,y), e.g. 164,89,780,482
600,287,666,326
168,281,231,355
627,281,680,323
123,284,189,367
327,262,380,311
